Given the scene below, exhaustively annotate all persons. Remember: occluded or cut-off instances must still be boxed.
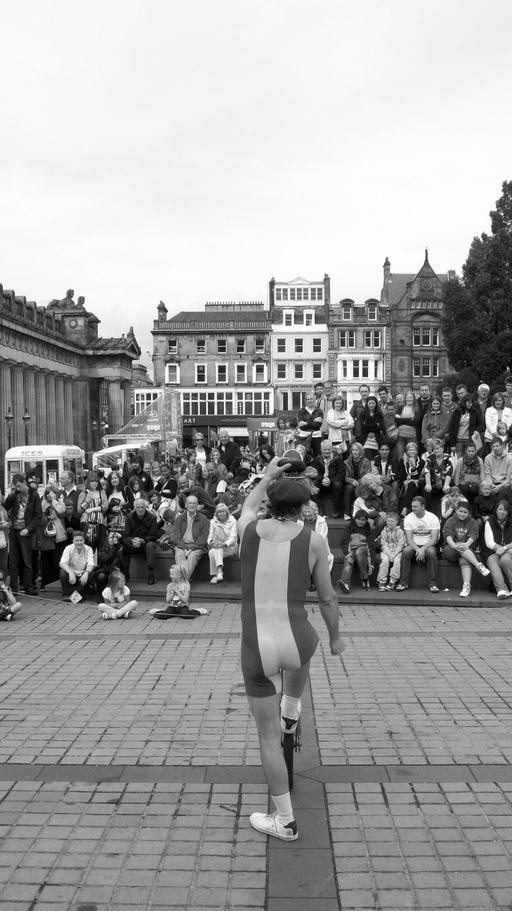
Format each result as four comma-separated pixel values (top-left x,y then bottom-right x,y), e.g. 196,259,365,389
377,383,399,419
361,473,399,537
151,461,161,488
395,391,422,458
106,471,136,532
381,400,402,436
421,396,449,452
230,453,348,842
238,444,281,478
442,501,492,598
122,498,156,584
202,447,233,498
493,423,512,456
94,530,132,603
215,430,241,480
503,376,512,408
349,384,383,444
219,480,244,520
396,495,442,592
147,563,210,620
376,511,406,593
473,482,500,518
154,464,178,516
308,439,346,519
123,475,149,499
440,386,460,437
440,486,470,526
124,455,154,492
206,502,239,584
300,500,335,592
274,416,287,457
142,462,151,475
370,443,402,498
2,473,42,596
296,392,324,459
399,441,432,516
455,383,469,406
416,383,437,418
283,416,298,451
421,437,434,463
111,465,120,471
484,391,512,453
474,382,493,428
343,442,371,521
357,396,385,460
423,438,453,511
481,499,512,601
188,431,214,489
314,383,324,405
319,381,335,436
0,571,23,621
76,470,108,553
0,489,12,571
450,394,486,457
352,485,376,529
180,450,203,483
40,485,68,549
327,395,355,446
59,530,95,604
97,566,139,620
178,475,216,519
173,495,210,584
157,509,179,551
453,442,485,503
337,509,377,595
57,471,83,530
484,436,512,493
148,489,168,519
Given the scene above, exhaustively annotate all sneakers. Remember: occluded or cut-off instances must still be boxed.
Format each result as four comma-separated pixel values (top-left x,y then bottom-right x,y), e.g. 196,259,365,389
429,585,440,593
102,613,112,619
336,578,351,594
475,562,491,577
385,582,395,591
150,609,162,616
199,607,211,616
280,696,302,734
123,611,131,618
395,584,409,592
359,579,371,591
209,576,218,584
249,810,299,842
217,571,223,581
496,590,510,600
378,583,387,592
459,582,471,598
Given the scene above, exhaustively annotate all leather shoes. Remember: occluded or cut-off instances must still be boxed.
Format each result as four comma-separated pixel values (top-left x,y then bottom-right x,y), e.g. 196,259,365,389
146,575,155,585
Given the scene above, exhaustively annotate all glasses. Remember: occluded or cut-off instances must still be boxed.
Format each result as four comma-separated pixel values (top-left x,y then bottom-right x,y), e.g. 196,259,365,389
195,439,204,441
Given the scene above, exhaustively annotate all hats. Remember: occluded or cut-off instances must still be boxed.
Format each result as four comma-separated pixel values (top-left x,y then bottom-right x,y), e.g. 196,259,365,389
265,480,312,510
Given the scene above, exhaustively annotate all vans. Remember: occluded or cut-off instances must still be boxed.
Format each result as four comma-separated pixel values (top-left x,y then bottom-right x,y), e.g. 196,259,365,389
4,443,85,508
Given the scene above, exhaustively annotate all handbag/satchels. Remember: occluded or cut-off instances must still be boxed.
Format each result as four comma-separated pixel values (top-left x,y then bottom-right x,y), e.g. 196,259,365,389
43,520,56,537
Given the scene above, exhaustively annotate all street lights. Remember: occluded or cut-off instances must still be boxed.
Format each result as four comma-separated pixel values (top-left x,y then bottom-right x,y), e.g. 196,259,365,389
23,408,31,446
5,407,14,449
